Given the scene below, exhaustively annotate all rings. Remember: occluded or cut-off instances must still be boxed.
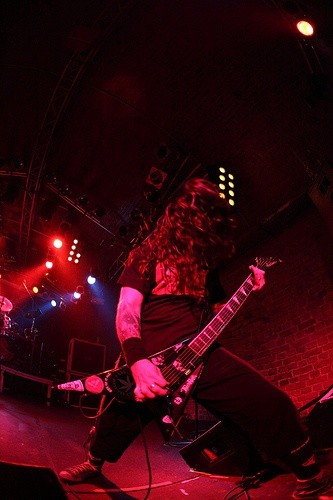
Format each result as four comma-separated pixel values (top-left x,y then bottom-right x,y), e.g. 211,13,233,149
149,384,155,391
258,277,261,281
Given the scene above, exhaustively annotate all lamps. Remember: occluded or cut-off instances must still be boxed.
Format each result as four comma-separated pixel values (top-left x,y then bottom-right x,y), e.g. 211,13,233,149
88,266,97,284
51,298,61,308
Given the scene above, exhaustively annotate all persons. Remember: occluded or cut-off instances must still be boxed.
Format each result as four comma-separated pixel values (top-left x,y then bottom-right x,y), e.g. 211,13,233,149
60,178,333,500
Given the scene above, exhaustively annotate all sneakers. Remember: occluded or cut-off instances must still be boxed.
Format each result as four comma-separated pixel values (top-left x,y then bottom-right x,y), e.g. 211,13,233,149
60,457,105,483
296,467,333,500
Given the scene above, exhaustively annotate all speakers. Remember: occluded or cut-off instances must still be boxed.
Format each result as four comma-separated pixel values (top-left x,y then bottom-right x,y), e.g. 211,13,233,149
180,420,263,477
0,461,69,500
304,387,333,450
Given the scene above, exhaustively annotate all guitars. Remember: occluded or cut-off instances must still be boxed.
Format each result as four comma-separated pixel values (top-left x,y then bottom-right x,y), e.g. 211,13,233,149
47,250,284,437
